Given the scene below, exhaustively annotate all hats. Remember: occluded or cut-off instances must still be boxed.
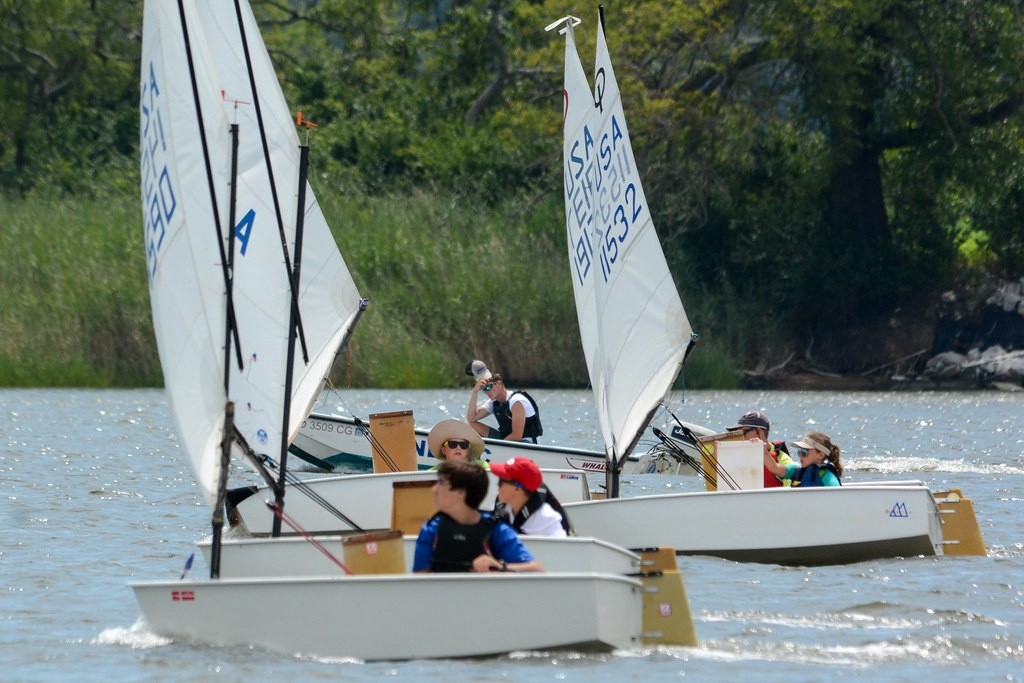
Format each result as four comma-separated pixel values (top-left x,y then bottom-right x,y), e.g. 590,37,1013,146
465,360,492,381
791,437,831,456
428,419,485,461
489,457,542,493
726,411,769,431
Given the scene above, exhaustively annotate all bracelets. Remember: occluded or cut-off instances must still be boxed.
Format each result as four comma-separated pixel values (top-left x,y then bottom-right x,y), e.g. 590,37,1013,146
498,559,506,571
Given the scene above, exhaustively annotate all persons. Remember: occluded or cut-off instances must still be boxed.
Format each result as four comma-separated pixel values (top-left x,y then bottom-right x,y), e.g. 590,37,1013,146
423,418,491,470
488,457,569,538
748,430,843,487
725,411,801,488
465,372,542,444
410,459,541,576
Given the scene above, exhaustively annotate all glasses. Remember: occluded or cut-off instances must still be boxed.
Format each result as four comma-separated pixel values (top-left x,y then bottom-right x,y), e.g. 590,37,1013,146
442,441,469,449
743,427,756,435
498,478,519,487
798,449,815,457
482,381,498,392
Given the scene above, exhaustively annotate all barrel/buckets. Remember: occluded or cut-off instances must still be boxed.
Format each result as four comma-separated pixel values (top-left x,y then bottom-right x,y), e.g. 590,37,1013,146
392,480,441,536
343,528,406,577
714,441,766,491
368,409,419,474
698,429,746,491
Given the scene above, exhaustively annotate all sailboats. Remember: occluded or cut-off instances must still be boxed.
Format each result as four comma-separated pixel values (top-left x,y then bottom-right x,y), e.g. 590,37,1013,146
127,0,962,663
